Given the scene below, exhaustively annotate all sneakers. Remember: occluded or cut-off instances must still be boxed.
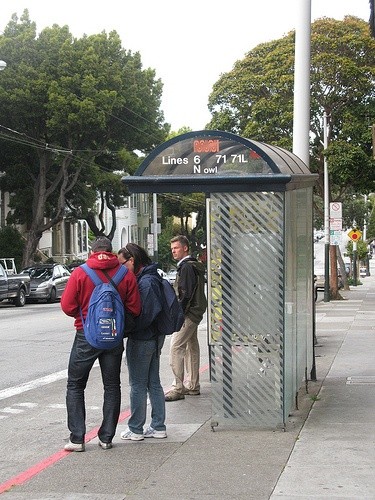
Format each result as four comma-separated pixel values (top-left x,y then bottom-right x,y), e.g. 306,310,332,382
182,387,200,395
64,440,84,452
144,426,167,438
164,390,185,401
98,440,113,449
121,427,144,441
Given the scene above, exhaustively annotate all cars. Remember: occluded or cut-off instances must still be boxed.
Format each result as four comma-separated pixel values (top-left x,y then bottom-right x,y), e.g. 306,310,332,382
23,262,71,303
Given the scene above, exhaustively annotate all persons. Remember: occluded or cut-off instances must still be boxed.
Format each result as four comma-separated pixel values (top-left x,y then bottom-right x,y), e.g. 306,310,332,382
164,235,207,401
60,237,140,451
117,243,168,442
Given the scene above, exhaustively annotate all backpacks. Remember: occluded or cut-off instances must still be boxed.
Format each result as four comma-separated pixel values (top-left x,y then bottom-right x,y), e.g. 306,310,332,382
79,262,128,350
138,270,184,334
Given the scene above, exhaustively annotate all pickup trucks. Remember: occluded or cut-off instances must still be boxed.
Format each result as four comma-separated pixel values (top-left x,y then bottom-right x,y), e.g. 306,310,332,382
0,264,32,307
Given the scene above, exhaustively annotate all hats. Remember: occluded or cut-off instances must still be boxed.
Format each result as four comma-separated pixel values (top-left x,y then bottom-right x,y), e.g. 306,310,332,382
93,237,112,252
